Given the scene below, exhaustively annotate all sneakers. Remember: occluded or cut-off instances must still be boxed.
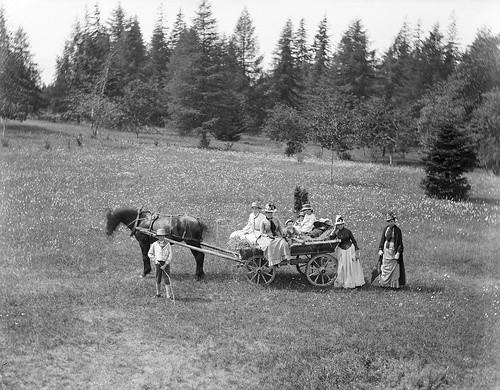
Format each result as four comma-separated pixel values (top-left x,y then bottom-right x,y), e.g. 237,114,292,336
166,297,174,303
153,294,163,299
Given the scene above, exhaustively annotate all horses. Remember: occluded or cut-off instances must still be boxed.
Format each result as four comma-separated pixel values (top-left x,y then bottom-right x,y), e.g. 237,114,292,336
105,208,209,282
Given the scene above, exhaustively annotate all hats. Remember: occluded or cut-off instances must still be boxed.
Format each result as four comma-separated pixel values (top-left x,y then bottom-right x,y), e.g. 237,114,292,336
264,203,276,213
301,204,311,211
386,212,396,221
334,215,344,225
299,212,305,216
251,202,262,210
285,219,293,226
156,228,166,236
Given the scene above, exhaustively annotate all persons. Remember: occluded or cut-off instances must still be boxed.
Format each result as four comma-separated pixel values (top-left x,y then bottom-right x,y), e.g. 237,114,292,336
330,216,365,289
294,202,316,234
295,212,305,225
228,201,265,249
370,209,405,290
259,202,291,268
283,219,306,248
147,228,172,300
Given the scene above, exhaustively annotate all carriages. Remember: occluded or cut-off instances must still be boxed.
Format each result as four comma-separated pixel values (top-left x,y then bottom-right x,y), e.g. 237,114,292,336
105,207,343,288
